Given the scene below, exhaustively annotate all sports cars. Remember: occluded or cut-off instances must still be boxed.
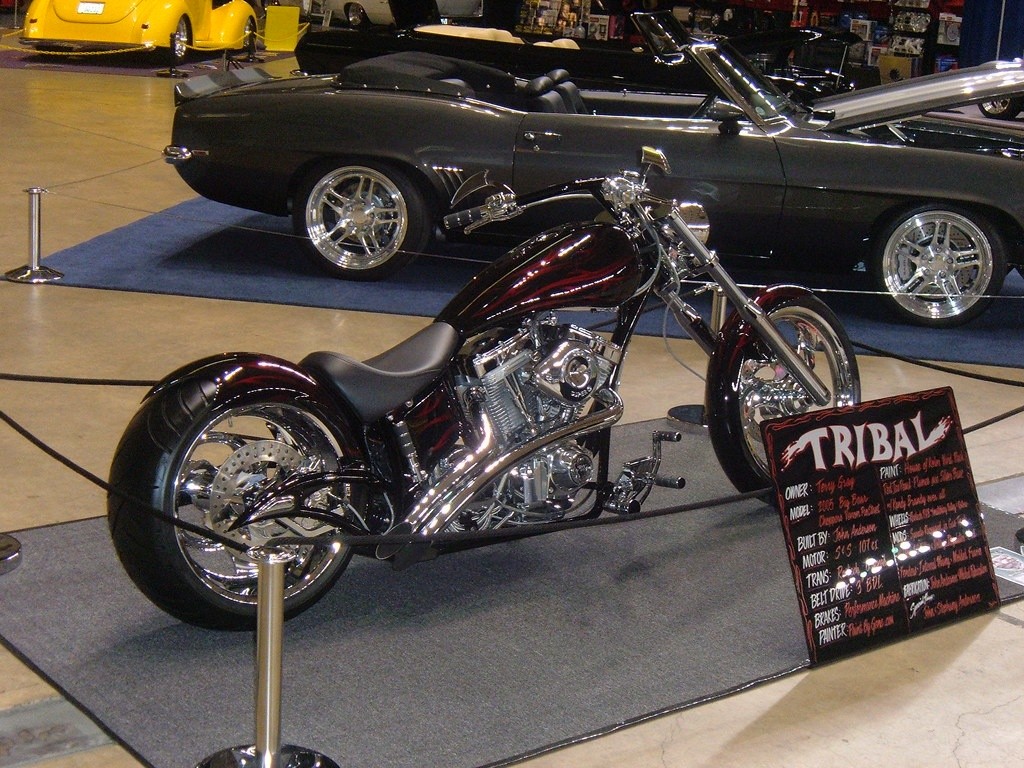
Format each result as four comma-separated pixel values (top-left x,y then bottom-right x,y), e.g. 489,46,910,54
18,0,259,61
161,7,1024,320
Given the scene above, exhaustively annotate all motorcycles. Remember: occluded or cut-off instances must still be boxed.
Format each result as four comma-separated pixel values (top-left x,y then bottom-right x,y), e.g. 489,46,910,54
105,145,857,634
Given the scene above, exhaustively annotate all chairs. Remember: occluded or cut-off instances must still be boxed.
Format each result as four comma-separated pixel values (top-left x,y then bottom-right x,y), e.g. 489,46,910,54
523,69,590,114
533,39,580,49
